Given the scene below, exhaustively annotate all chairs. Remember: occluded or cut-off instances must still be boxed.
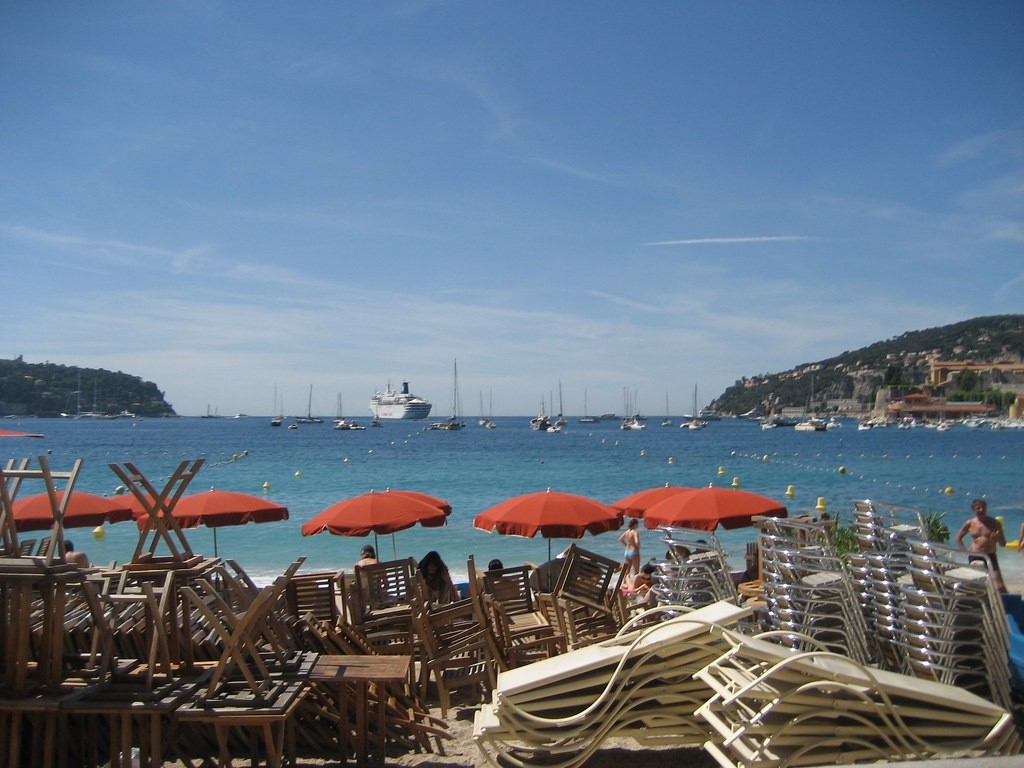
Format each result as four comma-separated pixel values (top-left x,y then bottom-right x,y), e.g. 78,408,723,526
0,453,1024,768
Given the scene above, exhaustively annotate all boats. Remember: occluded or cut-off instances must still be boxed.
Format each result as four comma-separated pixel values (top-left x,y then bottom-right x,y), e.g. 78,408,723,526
369,381,434,420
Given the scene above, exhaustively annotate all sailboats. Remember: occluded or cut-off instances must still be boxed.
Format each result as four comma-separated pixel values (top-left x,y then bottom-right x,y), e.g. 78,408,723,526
60,354,1023,434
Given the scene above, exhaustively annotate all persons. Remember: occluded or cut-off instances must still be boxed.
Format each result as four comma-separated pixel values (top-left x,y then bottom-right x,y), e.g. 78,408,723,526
954,499,1007,594
1017,524,1024,552
353,544,389,602
59,540,90,568
488,560,520,602
630,562,659,610
691,540,715,572
618,518,640,584
417,551,460,605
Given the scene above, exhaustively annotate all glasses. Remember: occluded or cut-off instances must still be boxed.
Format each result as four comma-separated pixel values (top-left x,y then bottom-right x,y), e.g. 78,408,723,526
641,575,651,580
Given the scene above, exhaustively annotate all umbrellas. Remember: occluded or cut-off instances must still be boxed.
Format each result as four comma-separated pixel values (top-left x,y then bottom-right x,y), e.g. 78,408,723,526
472,486,625,594
609,481,789,536
136,485,290,593
301,486,453,592
12,486,171,537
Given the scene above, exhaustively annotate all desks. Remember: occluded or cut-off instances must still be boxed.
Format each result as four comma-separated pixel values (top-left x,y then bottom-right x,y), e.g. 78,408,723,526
0,678,108,768
118,659,222,768
59,679,198,768
45,656,141,768
175,684,311,768
307,654,412,768
208,650,320,768
